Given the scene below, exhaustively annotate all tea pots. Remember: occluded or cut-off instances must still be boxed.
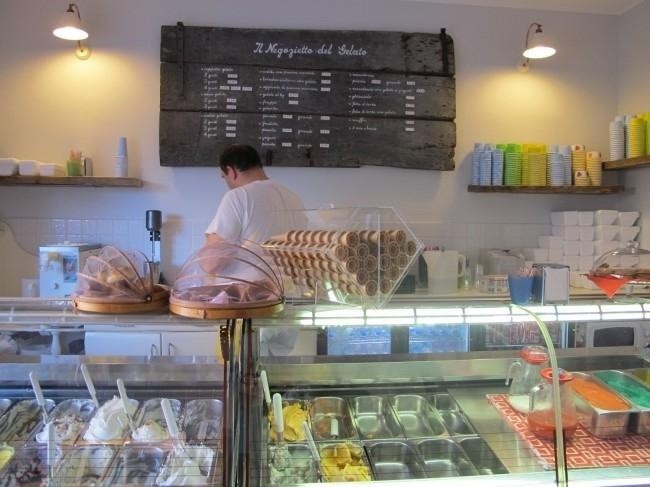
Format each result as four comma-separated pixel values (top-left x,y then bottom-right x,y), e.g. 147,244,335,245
505,346,550,414
528,365,578,439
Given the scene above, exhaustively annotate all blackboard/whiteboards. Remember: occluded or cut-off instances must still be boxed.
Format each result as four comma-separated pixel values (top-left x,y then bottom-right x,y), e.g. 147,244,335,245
159,25,456,171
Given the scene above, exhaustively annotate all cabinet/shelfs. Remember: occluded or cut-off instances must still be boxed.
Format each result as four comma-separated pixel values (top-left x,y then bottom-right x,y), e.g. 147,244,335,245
466,154,650,194
0,296,650,487
82,324,318,359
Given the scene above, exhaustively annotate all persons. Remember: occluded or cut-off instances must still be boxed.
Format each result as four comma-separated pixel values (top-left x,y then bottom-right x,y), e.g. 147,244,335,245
63,256,77,281
198,145,308,282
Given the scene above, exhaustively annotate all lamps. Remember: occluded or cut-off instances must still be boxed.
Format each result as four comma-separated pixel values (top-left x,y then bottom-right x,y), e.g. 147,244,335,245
517,22,556,72
52,3,90,60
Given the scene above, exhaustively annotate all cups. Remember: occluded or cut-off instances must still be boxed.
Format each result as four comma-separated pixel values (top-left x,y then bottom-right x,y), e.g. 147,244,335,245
421,249,466,294
66,159,83,175
508,274,535,306
21,276,39,297
114,136,129,178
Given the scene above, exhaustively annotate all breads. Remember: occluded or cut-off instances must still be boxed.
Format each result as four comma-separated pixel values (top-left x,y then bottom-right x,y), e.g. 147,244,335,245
97,265,137,284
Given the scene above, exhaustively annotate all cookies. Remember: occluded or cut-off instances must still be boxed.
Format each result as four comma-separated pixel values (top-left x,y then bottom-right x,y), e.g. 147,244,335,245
595,269,650,279
